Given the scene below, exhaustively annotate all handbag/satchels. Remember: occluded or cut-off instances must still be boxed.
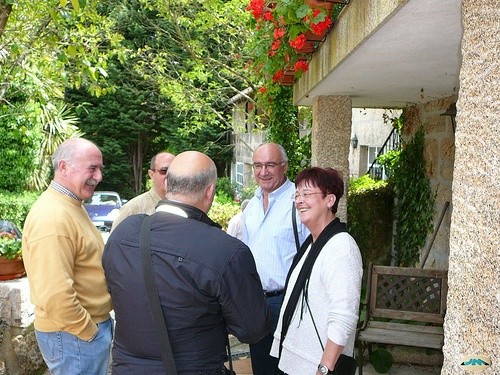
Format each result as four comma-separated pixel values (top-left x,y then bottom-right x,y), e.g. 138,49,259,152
328,354,356,375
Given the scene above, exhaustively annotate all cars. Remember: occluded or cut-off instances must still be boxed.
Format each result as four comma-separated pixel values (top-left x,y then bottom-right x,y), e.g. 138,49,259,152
81,190,128,232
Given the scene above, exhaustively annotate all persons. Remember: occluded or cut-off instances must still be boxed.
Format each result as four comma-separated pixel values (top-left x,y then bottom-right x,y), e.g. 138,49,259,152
269,166,363,375
225,200,249,245
21,138,113,375
240,142,311,374
112,153,176,232
101,151,271,375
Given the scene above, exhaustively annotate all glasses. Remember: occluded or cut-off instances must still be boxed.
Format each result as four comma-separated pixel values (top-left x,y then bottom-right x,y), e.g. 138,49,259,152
155,169,167,174
253,161,284,169
291,192,331,202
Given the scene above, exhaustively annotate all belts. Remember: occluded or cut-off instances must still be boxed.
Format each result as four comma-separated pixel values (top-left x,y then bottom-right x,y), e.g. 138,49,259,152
263,288,285,298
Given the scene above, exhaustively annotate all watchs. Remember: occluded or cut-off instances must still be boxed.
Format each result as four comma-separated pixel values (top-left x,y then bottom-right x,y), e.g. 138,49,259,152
316,361,338,375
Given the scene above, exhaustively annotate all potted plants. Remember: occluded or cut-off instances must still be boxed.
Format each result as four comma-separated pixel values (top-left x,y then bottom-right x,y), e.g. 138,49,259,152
0,228,26,282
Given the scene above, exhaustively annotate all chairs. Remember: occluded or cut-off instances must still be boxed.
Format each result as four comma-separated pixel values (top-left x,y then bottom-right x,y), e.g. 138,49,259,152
353,262,448,375
